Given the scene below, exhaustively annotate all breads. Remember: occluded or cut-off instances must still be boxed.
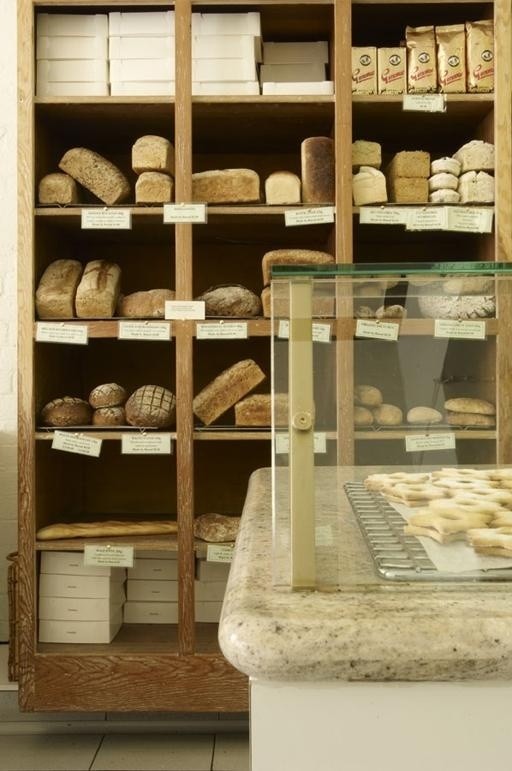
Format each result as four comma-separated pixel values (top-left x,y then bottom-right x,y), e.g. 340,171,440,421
35,135,512,559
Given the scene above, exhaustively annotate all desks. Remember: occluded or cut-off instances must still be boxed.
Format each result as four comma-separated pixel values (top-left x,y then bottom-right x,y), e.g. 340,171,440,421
217,465,510,770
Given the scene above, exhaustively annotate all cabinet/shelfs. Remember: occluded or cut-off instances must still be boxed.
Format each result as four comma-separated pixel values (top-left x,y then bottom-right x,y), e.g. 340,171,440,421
16,0,512,719
